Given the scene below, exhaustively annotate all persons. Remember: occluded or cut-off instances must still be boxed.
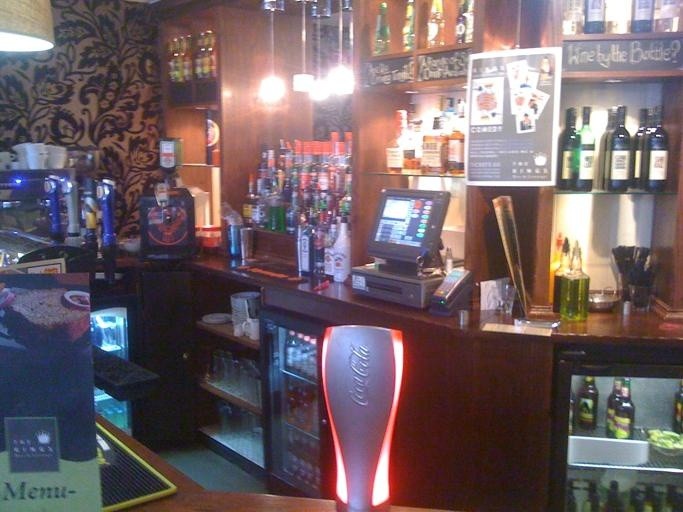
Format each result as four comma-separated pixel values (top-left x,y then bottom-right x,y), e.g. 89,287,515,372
541,55,551,77
521,77,532,88
520,113,532,131
529,94,539,114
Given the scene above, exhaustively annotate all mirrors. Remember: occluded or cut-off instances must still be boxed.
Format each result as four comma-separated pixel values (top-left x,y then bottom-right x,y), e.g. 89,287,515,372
311,1,351,143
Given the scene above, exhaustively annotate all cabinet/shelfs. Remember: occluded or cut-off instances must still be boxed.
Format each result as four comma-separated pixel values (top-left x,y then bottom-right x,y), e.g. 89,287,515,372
353,1,464,180
271,361,321,499
190,260,272,484
553,0,682,341
158,1,315,256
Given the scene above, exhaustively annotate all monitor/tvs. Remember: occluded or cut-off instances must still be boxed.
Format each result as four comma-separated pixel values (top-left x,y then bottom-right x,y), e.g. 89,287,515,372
366,188,450,275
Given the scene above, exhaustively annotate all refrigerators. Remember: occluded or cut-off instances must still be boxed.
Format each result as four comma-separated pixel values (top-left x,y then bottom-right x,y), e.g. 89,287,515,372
255,306,334,500
547,344,683,511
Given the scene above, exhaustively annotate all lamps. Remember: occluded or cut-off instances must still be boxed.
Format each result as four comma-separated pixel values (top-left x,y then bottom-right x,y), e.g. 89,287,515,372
291,4,316,96
0,0,55,64
310,0,333,102
326,0,351,97
257,1,290,110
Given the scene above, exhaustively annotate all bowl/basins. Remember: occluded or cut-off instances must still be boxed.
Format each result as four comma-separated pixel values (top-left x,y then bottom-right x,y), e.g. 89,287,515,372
588,289,622,311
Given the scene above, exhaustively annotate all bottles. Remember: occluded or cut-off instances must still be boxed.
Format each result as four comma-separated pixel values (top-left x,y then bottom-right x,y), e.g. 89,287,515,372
549,230,589,325
569,374,635,439
371,1,474,58
443,246,453,274
240,128,351,286
165,30,216,83
280,329,320,487
567,475,683,512
382,96,464,175
562,1,683,35
558,104,671,195
672,379,683,434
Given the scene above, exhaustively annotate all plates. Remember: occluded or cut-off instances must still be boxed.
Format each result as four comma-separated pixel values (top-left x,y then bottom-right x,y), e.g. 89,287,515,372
198,289,260,337
62,290,90,309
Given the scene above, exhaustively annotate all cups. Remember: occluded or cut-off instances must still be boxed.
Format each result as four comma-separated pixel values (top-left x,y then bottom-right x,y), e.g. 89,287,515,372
0,142,66,171
241,317,261,342
211,349,263,404
617,272,631,303
628,284,656,312
215,405,261,463
226,223,243,258
